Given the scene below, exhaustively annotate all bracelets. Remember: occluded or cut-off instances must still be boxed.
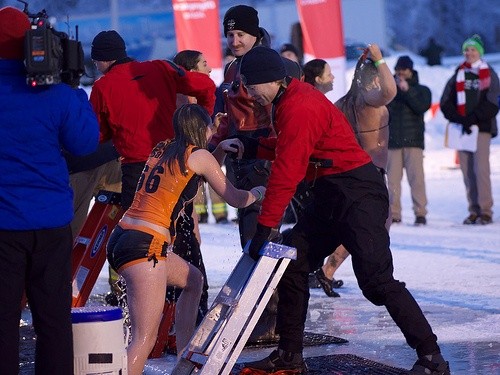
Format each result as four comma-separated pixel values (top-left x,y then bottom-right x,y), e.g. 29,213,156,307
257,189,262,201
374,59,385,67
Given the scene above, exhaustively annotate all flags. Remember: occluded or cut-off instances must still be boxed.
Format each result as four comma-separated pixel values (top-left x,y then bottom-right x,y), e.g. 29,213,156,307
297,0,346,104
172,0,225,86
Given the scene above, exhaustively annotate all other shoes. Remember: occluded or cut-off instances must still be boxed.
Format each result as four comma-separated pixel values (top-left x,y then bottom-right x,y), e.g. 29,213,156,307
309,267,344,298
198,212,229,224
409,349,451,375
463,214,492,225
241,348,308,375
415,216,426,225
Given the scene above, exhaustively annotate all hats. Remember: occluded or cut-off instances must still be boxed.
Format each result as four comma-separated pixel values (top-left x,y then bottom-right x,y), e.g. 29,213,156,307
223,5,260,39
241,46,291,86
462,35,484,57
395,56,413,71
90,30,126,62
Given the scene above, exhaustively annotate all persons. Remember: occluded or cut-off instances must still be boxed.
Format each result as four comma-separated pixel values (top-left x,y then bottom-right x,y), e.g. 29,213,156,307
0,5,99,375
223,5,302,342
173,50,212,106
240,46,451,375
317,44,397,297
88,30,216,358
107,103,266,375
424,38,441,66
281,43,302,64
440,35,500,225
303,59,335,93
385,56,431,225
